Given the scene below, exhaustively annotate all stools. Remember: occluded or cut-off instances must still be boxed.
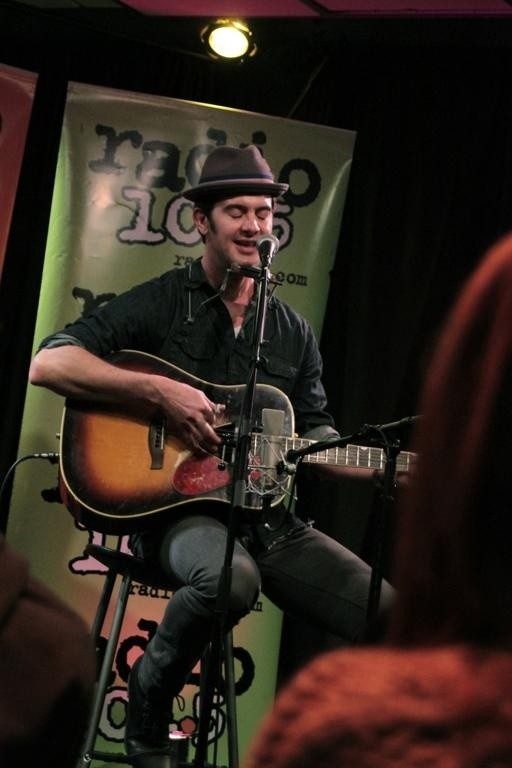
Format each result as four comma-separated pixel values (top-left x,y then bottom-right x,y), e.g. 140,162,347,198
75,544,238,765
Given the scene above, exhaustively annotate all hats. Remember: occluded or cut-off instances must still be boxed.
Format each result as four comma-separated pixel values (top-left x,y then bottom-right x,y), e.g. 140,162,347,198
183,144,289,202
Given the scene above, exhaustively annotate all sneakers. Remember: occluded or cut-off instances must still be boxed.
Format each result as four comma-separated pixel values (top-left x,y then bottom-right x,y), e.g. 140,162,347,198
125,654,185,768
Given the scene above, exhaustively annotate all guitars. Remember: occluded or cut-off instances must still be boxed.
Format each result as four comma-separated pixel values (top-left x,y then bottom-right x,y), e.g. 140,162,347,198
60,348,420,536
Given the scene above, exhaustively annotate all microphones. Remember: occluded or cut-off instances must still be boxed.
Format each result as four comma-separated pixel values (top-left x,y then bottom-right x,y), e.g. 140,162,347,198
256,234,279,269
260,408,285,511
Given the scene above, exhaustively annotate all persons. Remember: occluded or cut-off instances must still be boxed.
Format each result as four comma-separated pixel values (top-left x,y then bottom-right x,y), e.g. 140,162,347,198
29,143,396,765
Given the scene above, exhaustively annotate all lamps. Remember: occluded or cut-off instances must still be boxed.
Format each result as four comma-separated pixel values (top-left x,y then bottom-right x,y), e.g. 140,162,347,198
200,17,258,65
